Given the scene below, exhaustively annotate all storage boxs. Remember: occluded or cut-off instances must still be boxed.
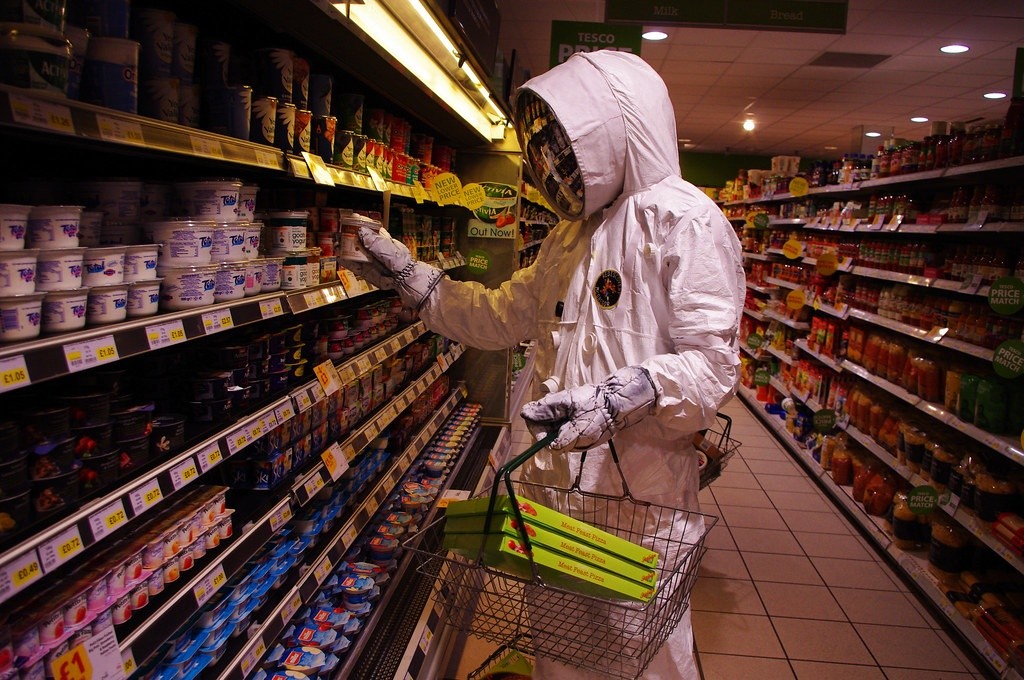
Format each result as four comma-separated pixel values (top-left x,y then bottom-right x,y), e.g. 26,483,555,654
443,0,501,75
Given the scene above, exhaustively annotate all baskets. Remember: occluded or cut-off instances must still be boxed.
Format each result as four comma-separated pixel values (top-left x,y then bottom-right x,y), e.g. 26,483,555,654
694,410,742,492
404,416,720,680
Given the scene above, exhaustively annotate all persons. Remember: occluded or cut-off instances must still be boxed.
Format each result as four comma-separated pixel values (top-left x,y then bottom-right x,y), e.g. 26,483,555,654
337,49,746,680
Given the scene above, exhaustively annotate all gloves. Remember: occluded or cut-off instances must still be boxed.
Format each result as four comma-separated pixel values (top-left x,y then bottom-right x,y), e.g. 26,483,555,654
521,364,658,453
330,213,445,320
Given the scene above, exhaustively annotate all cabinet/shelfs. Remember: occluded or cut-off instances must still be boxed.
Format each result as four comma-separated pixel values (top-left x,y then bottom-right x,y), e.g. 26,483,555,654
509,161,562,424
715,151,1024,680
0,0,509,679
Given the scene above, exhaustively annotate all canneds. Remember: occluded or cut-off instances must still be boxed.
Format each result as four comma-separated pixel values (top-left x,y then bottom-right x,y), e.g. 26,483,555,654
0,0,458,188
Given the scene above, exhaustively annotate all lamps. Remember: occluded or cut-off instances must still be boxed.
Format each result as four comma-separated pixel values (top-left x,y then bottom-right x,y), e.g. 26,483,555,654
378,0,513,129
